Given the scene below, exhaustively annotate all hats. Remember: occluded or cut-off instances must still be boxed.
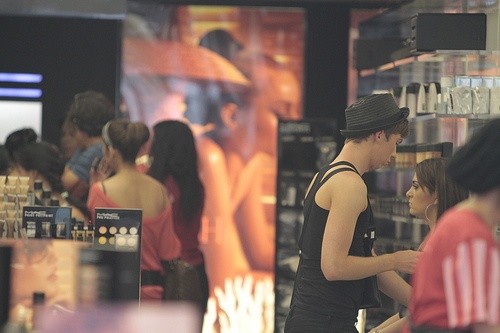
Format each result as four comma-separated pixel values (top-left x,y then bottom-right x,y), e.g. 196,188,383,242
339,92,410,134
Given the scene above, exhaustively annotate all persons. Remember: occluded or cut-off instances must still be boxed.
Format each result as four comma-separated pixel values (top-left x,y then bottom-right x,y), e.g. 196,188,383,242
0,88,210,332
120,18,251,295
186,29,301,290
409,119,500,333
285,93,422,333
367,158,445,333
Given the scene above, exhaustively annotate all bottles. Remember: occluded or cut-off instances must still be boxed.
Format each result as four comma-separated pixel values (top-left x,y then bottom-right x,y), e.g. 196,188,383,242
33,180,59,208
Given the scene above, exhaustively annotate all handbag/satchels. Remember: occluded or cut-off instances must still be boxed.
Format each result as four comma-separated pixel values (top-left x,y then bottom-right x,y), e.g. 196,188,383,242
360,246,383,309
